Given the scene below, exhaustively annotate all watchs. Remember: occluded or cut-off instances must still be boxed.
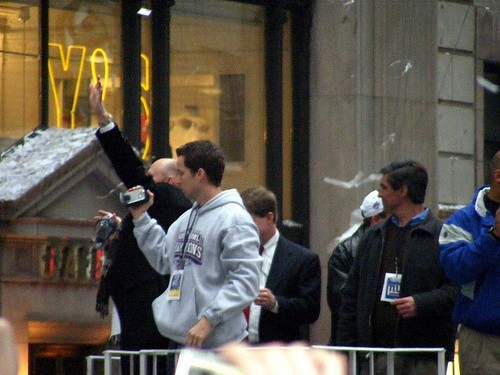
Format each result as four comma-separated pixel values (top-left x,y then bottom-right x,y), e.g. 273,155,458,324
488,227,500,242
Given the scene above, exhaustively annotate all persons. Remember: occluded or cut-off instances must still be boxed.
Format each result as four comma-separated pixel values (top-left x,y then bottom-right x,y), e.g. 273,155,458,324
340,159,456,375
326,189,389,349
437,149,500,375
239,186,323,346
125,139,261,351
87,75,192,375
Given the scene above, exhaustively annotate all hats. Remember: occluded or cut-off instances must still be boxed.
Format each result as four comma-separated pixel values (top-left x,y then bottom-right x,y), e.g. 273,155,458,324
359,190,384,218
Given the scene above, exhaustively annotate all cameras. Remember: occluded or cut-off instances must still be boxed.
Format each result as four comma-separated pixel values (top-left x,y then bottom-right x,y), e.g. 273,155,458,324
119,188,149,207
94,214,117,243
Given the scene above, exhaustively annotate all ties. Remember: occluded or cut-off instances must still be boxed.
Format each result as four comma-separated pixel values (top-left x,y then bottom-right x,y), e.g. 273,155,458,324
244,245,264,342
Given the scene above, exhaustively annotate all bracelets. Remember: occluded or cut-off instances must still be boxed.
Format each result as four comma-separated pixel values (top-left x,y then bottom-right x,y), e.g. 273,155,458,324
98,113,113,129
